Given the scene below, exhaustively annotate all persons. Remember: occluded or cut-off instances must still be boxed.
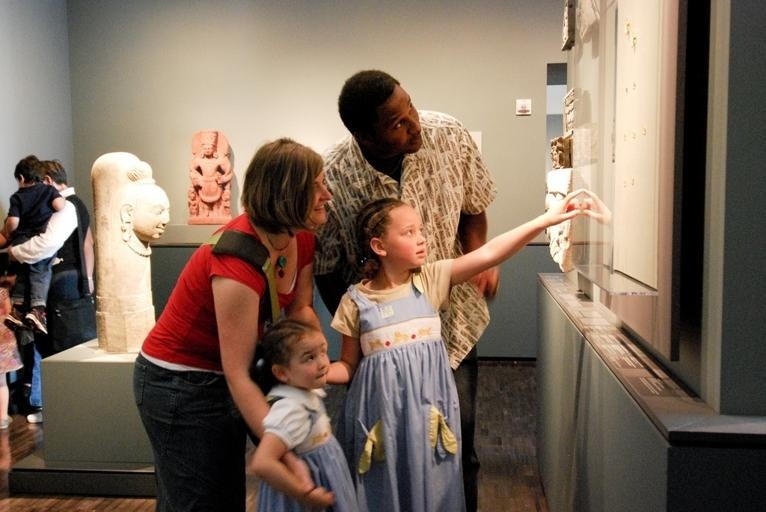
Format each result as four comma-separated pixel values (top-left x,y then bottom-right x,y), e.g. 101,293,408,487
0,154,99,430
316,187,585,512
250,318,362,511
119,161,171,255
189,132,231,219
130,136,333,511
311,67,500,511
583,190,613,226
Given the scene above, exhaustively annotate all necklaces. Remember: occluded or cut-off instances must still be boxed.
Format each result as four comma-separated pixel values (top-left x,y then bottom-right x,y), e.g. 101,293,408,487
263,229,293,279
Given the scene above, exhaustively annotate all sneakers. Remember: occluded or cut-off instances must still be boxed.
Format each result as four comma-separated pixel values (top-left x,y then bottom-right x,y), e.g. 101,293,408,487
0,416,13,428
27,412,43,423
4,309,47,334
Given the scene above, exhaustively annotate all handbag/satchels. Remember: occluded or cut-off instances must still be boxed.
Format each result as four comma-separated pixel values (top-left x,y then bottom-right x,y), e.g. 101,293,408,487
47,296,94,327
